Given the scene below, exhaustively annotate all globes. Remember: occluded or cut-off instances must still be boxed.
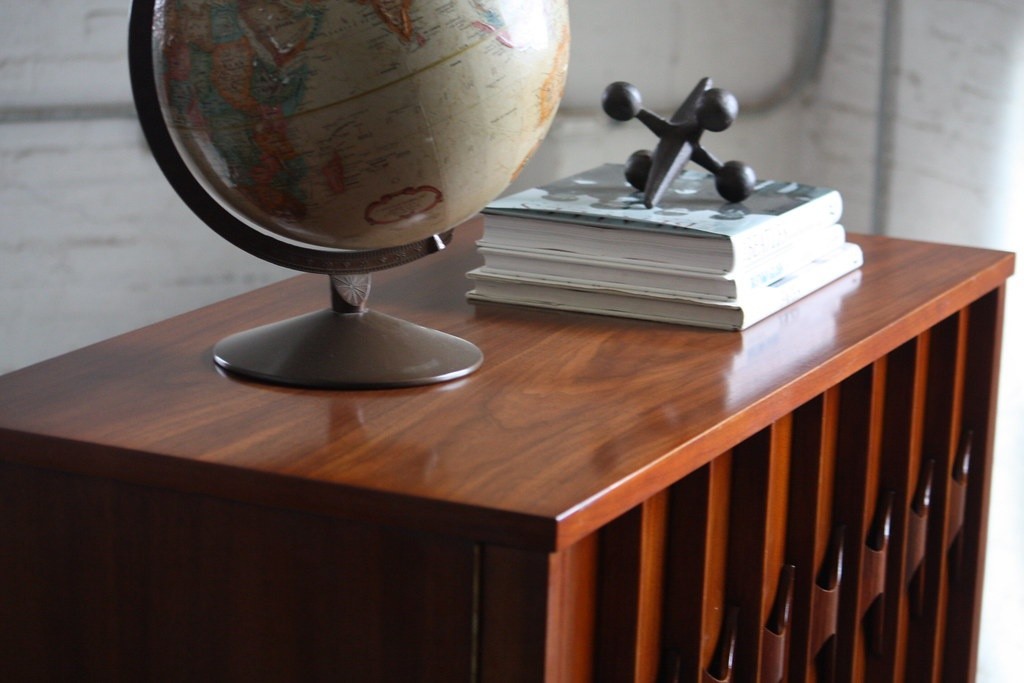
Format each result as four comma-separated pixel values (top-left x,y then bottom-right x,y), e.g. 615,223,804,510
128,0,570,393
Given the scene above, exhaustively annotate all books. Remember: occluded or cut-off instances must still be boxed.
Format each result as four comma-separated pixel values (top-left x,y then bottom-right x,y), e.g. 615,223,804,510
468,160,864,332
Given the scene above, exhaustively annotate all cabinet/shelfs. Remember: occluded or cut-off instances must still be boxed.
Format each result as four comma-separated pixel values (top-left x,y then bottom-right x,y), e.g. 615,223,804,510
1,230,1015,683
